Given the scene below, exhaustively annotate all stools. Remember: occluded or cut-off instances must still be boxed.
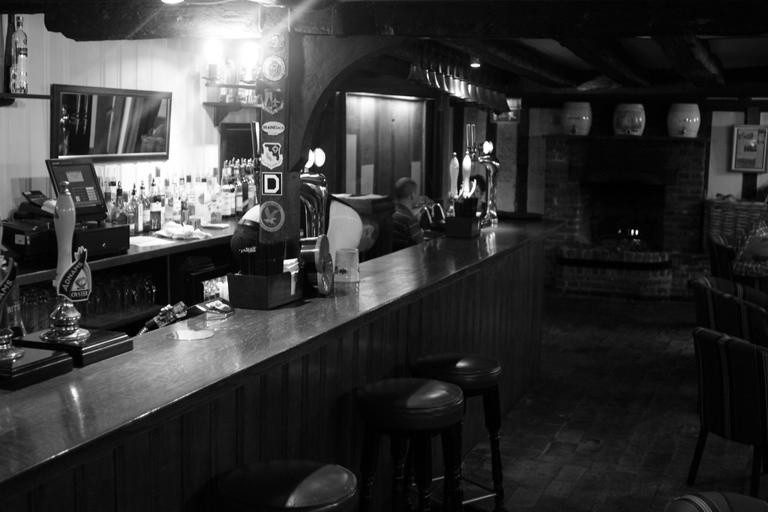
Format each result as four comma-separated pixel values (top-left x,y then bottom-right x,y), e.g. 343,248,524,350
351,378,466,512
408,353,505,512
217,459,360,512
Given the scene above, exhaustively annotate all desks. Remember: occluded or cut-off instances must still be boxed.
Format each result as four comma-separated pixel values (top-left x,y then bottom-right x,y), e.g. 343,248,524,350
7,214,240,337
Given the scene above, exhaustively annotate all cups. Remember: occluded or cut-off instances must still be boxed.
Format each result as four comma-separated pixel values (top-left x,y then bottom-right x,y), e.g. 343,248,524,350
333,247,360,283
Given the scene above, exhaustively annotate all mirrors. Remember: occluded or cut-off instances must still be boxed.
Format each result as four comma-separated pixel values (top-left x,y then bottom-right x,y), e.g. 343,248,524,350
50,83,172,163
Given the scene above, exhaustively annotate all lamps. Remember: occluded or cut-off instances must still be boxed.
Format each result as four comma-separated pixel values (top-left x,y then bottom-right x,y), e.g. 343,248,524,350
470,53,480,68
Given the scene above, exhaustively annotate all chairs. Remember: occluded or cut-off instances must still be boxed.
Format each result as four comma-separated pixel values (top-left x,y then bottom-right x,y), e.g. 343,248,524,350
664,230,768,512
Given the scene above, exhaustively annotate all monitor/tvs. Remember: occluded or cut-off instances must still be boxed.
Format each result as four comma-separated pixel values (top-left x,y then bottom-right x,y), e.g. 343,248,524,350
45,158,108,216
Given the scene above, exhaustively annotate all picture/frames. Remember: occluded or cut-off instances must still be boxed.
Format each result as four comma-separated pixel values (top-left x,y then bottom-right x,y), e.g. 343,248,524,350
728,123,768,171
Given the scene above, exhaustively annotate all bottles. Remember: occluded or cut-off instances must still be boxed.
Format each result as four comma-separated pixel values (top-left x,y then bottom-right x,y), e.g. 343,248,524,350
300,234,334,298
449,150,472,197
7,15,29,95
0,261,23,362
53,178,78,288
105,155,258,236
218,87,234,104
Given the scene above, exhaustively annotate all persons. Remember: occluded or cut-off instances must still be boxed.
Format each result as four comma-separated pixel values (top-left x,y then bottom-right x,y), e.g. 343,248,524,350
469,173,487,215
388,174,425,250
226,204,262,273
